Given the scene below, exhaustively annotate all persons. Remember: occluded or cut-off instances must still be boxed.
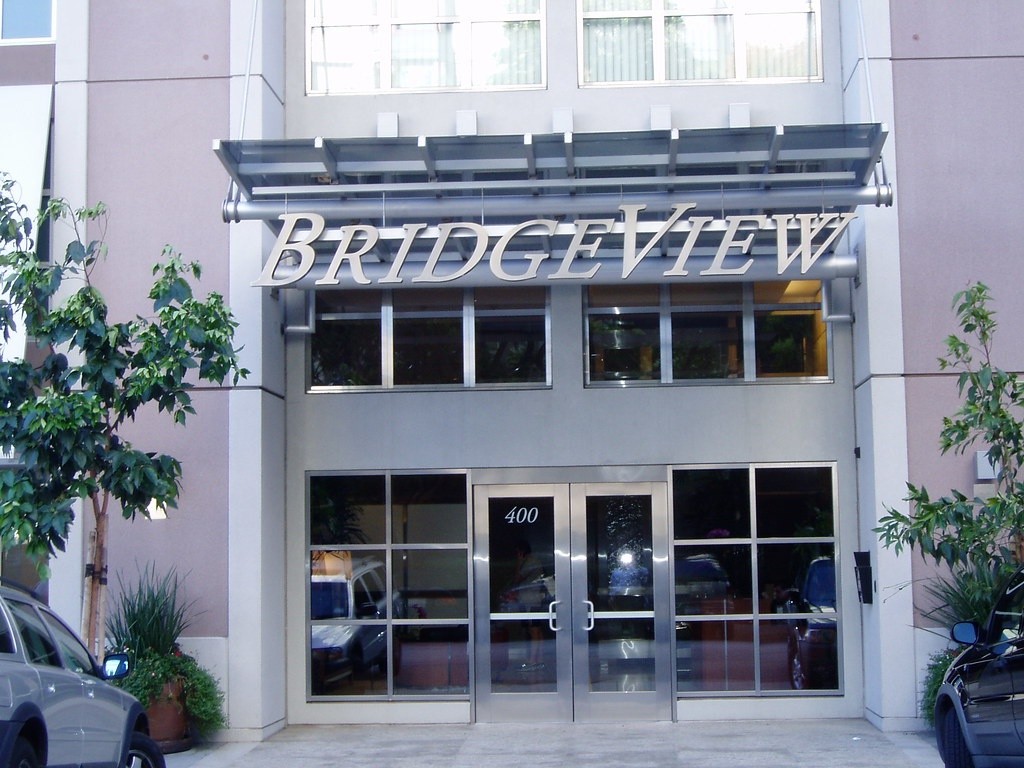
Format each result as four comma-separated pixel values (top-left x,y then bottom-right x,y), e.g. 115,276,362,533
515,541,546,669
609,542,648,609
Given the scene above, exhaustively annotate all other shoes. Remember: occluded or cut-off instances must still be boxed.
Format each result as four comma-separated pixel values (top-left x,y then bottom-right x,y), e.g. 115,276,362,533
517,664,537,672
536,663,546,672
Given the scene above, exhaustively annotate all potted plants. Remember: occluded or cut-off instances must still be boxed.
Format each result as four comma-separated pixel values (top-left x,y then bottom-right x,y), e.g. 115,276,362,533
80,553,231,754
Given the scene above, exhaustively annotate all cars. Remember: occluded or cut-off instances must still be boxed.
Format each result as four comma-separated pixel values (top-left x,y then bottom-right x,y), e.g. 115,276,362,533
782,554,838,691
496,554,558,617
933,560,1024,768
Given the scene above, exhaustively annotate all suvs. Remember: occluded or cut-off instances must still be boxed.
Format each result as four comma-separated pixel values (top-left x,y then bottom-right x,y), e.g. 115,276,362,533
310,556,405,686
0,582,166,768
